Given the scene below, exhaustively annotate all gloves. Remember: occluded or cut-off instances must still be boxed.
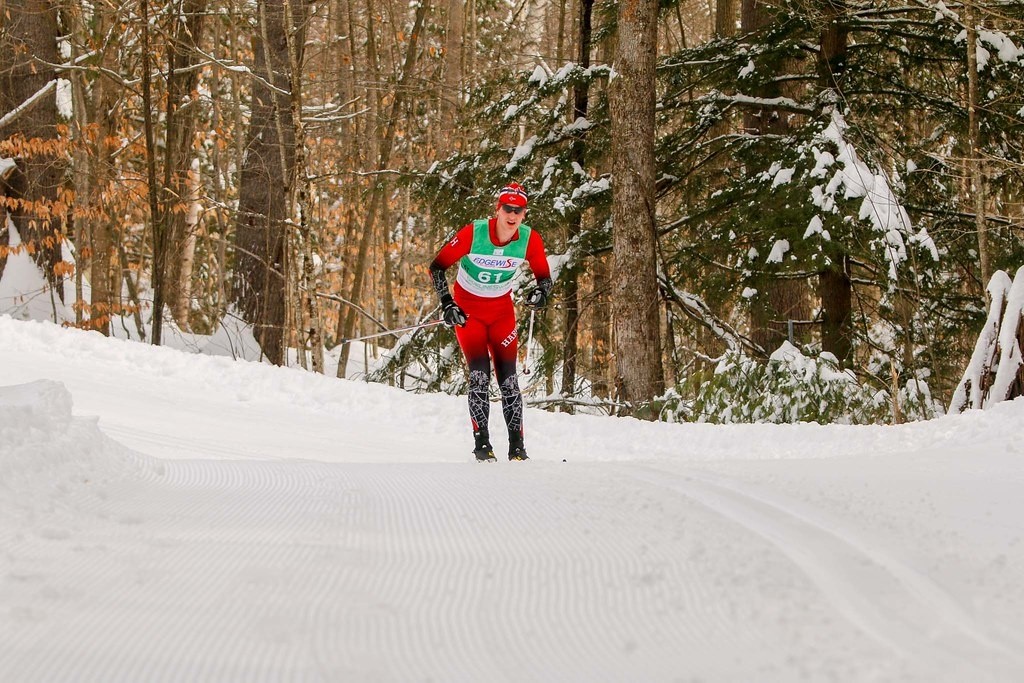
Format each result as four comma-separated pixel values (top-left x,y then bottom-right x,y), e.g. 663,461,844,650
526,286,550,311
441,294,467,328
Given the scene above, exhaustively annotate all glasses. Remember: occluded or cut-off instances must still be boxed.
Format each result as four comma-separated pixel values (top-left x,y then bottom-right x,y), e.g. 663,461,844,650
502,205,524,215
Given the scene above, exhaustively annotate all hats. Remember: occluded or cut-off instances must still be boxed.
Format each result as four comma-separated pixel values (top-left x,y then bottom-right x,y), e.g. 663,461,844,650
499,183,528,208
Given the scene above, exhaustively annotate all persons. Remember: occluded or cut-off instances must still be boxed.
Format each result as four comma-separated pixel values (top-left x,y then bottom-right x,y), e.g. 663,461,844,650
429,183,554,461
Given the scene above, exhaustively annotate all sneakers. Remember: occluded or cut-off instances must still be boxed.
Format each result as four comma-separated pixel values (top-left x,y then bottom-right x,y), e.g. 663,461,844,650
508,440,531,462
472,429,498,464
